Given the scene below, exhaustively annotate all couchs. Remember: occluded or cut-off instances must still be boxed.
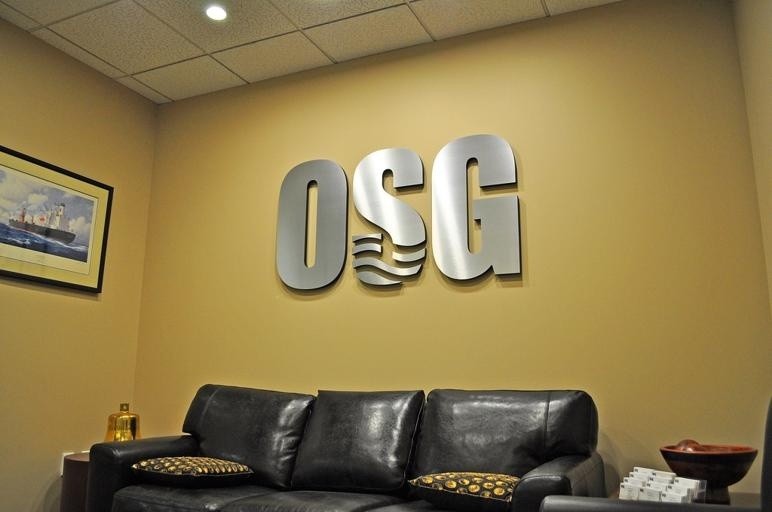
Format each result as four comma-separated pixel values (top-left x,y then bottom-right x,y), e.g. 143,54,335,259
89,384,604,512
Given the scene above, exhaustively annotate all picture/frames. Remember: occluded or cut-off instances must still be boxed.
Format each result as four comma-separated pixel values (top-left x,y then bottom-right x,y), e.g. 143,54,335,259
0,145,114,294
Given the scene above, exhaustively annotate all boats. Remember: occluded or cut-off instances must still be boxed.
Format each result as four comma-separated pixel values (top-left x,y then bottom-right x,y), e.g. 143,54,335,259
9,203,76,245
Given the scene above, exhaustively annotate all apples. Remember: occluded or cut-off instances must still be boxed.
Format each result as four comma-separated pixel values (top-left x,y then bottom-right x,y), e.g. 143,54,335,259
686,444,706,452
675,439,700,452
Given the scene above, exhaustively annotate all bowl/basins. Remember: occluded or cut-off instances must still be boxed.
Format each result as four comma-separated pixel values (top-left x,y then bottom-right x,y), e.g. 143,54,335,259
659,446,757,503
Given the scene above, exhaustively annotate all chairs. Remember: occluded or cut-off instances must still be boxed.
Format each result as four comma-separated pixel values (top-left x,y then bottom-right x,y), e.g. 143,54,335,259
541,398,772,512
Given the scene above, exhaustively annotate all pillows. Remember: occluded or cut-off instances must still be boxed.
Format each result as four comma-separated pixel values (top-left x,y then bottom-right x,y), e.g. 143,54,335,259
128,455,254,488
291,389,427,496
406,472,520,511
184,385,315,489
409,388,599,494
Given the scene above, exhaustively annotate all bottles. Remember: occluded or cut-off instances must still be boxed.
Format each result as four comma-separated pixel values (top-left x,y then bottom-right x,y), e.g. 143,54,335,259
103,403,143,444
